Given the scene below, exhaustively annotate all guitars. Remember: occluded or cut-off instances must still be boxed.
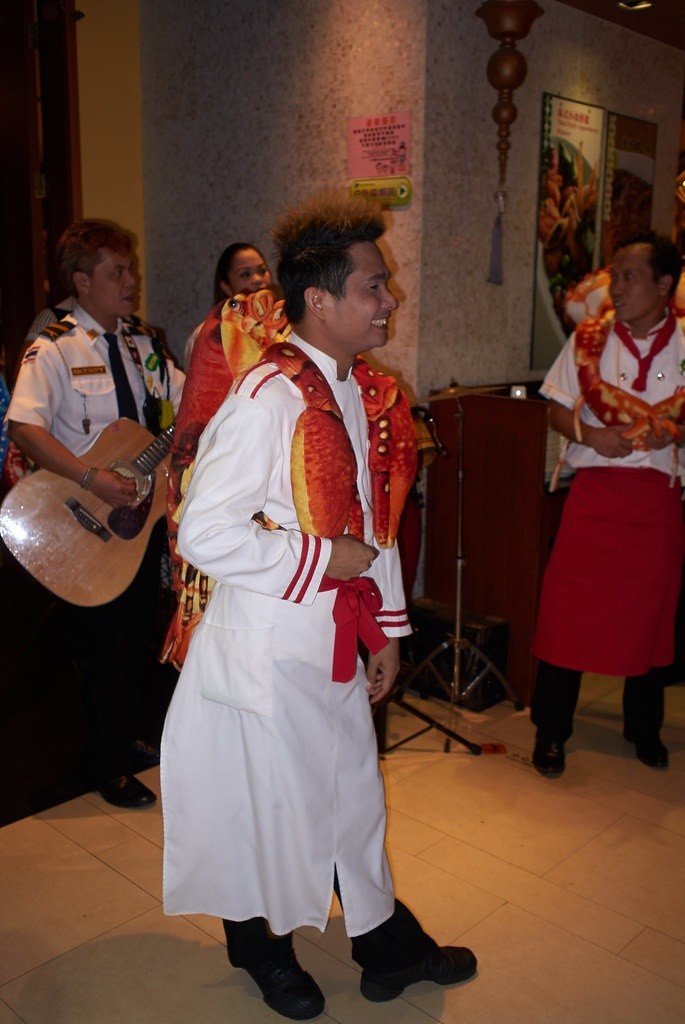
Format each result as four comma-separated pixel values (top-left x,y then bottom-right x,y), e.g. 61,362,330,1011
0,415,177,608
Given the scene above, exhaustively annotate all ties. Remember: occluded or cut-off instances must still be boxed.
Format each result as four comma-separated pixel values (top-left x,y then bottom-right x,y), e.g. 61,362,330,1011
103,333,140,425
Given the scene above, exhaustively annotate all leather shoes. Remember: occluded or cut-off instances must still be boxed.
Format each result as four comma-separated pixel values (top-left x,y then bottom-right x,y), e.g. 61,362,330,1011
360,946,478,1002
94,773,156,808
532,740,566,777
122,738,160,764
227,947,326,1020
623,734,668,767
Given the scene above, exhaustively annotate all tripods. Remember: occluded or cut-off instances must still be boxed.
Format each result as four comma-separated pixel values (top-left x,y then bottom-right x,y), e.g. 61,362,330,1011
373,695,482,757
401,396,523,711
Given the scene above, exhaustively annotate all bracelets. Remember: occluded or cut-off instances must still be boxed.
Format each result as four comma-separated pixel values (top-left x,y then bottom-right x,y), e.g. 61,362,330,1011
80,466,98,489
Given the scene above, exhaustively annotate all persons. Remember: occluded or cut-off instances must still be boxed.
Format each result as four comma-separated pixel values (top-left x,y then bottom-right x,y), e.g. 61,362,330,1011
3,226,186,808
184,242,272,375
159,196,478,1020
13,219,169,386
530,232,685,777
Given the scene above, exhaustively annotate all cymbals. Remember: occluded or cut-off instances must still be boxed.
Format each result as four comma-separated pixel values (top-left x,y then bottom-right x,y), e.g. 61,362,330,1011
407,385,505,403
